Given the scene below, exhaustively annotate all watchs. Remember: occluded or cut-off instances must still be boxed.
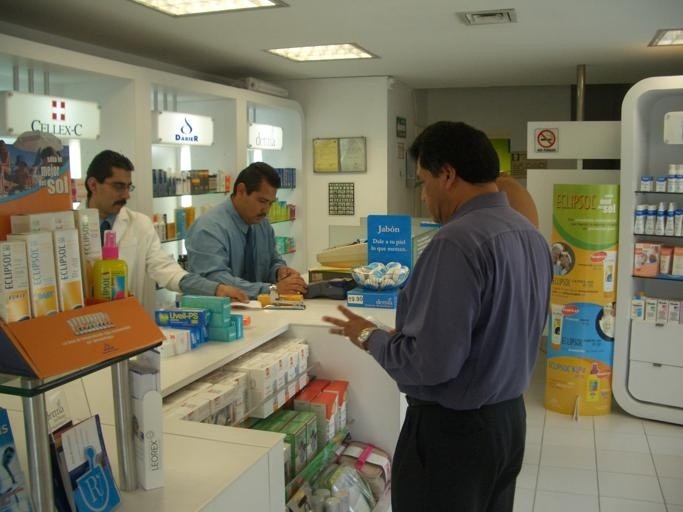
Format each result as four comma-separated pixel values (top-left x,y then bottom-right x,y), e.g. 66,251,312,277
357,326,380,350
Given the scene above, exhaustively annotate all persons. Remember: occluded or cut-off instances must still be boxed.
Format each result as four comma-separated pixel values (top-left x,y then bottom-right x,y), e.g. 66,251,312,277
321,120,553,511
549,242,572,276
12,147,63,191
184,162,308,300
70,150,249,314
496,168,539,230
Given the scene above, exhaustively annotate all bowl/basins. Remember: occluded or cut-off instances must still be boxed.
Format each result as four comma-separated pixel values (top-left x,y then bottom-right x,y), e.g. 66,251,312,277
351,263,410,291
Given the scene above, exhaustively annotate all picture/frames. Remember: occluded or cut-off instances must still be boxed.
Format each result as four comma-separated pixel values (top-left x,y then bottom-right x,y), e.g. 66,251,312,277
312,136,366,173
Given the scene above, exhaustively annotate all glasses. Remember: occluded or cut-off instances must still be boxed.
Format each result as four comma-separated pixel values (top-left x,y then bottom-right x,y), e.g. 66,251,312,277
104,181,134,194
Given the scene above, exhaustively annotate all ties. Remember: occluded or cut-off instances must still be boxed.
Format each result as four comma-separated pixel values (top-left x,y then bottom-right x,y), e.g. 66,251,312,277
243,226,256,282
100,220,110,246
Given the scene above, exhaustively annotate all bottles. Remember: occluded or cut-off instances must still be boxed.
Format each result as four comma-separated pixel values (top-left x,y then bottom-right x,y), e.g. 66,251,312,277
633,164,683,238
310,488,349,511
631,291,647,321
659,247,672,275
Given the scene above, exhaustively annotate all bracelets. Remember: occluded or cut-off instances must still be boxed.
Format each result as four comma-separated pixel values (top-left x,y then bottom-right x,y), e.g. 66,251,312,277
269,284,278,295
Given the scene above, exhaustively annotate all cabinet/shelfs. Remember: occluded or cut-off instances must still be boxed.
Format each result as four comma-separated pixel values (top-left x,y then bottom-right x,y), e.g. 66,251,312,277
611,75,682,426
0,33,308,308
